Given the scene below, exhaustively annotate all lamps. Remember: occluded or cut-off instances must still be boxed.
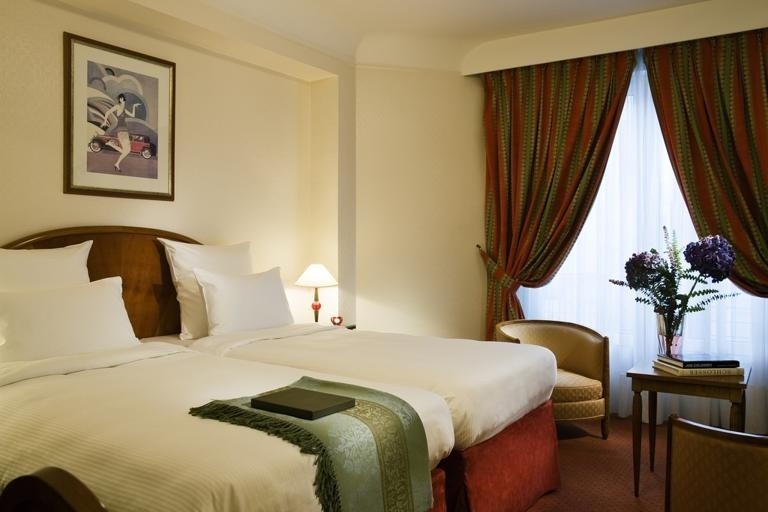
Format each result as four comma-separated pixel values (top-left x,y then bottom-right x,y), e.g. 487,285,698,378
290,259,342,326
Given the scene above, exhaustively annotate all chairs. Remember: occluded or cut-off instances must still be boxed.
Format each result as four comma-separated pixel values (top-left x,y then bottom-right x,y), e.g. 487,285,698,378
491,316,614,443
664,407,768,509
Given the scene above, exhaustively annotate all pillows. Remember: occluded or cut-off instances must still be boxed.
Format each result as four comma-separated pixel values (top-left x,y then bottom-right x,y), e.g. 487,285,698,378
0,237,97,304
151,231,253,341
0,275,157,385
192,263,295,342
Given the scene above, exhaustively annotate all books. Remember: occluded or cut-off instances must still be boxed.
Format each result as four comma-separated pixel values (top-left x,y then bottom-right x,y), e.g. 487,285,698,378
657,353,740,369
652,358,744,377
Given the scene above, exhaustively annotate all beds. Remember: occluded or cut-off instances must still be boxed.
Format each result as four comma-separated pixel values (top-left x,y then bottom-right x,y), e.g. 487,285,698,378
0,224,564,511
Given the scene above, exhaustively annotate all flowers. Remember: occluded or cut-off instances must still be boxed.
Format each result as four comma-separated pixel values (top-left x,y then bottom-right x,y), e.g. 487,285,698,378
603,221,745,360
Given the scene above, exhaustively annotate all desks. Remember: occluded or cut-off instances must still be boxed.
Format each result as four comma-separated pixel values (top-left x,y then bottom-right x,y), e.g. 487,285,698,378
624,351,755,504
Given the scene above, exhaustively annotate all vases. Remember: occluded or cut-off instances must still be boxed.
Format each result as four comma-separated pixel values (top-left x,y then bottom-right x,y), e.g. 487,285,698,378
654,310,684,361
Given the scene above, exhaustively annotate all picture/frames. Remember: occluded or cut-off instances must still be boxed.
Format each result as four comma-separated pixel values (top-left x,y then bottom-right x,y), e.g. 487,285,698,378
60,30,177,204
